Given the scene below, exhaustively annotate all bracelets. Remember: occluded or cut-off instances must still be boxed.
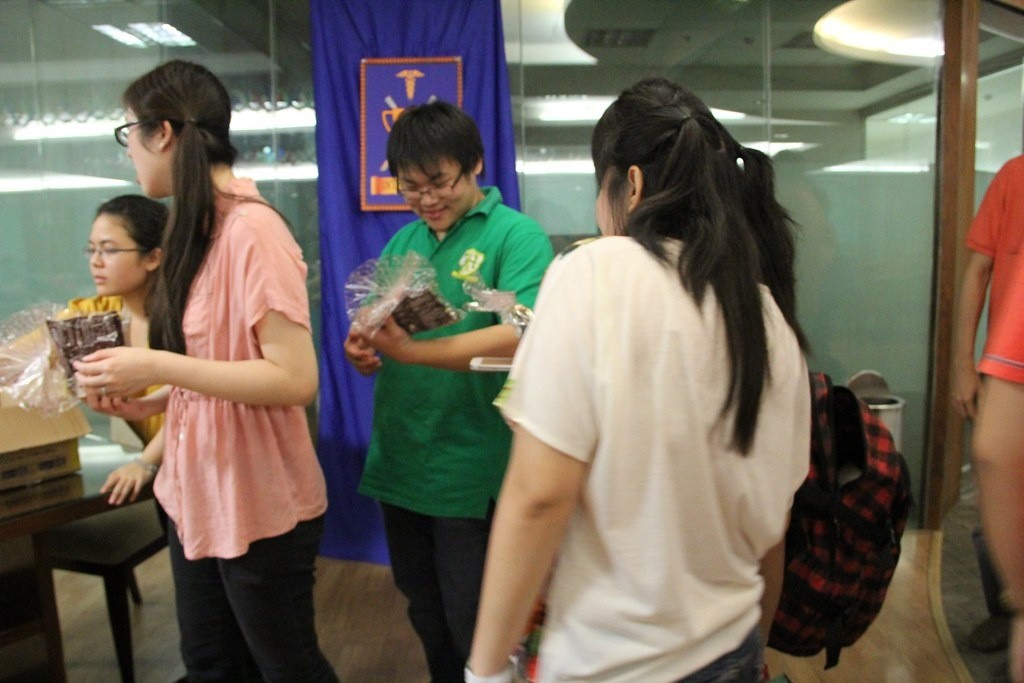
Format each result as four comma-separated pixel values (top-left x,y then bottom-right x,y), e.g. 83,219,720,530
134,457,159,471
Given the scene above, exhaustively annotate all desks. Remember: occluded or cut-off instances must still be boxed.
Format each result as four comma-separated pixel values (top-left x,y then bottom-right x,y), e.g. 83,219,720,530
0,433,147,683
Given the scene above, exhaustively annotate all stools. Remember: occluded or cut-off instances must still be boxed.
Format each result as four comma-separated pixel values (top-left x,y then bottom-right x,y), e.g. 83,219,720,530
33,502,175,683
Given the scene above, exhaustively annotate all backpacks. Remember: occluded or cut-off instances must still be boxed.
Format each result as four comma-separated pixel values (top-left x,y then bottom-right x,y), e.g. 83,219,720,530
767,373,915,671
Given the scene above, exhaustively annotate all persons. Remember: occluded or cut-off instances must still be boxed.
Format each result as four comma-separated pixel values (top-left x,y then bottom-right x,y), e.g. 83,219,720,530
0,194,170,538
948,154,1024,683
344,100,555,683
464,78,812,683
71,58,340,683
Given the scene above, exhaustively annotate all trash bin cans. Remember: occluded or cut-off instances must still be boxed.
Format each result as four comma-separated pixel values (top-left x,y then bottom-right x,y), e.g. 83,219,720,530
845,395,904,453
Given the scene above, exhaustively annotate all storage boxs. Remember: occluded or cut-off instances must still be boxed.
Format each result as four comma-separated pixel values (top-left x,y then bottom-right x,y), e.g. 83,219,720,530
0,393,95,491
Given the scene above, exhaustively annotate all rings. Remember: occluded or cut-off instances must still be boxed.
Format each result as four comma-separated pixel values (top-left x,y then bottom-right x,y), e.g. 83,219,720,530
103,386,108,397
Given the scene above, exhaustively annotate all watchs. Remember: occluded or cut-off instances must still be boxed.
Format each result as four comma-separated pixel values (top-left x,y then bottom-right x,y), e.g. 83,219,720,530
465,643,528,683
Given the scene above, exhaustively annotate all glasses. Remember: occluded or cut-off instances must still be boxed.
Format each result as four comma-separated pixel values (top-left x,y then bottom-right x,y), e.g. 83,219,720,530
396,162,467,201
115,121,144,148
81,242,151,261
